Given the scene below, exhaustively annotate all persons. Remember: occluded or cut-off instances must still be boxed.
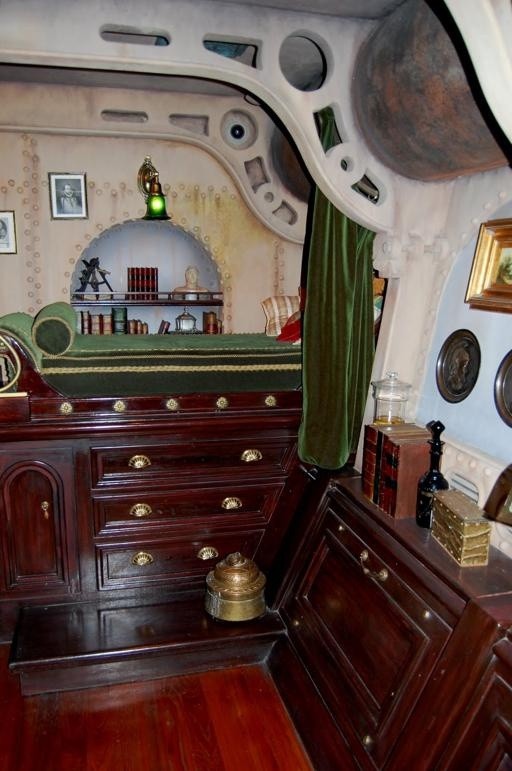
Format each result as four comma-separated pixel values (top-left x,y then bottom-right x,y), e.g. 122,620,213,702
173,266,211,292
60,183,80,214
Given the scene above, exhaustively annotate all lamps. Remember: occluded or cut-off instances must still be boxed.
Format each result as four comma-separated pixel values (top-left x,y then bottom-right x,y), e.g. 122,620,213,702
137,155,172,221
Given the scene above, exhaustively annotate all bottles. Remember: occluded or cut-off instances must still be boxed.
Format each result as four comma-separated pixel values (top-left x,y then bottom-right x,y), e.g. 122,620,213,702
414,421,450,528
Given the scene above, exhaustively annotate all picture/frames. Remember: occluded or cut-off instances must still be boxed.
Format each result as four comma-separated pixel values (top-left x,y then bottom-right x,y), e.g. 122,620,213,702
464,218,512,314
48,171,89,220
0,209,18,254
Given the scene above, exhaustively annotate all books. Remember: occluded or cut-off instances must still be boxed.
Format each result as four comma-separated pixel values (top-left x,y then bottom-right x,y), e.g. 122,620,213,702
361,423,433,519
157,319,171,334
74,307,148,335
126,267,159,300
201,310,223,335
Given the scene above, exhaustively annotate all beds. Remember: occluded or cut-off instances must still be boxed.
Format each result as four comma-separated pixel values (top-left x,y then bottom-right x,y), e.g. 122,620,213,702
0,287,305,395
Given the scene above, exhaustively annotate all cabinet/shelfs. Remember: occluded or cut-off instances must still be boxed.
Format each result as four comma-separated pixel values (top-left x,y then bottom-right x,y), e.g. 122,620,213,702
69,290,223,335
1,327,305,696
260,459,512,771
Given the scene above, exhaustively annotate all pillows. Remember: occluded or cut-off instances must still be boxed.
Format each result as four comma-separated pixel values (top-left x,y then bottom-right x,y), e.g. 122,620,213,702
276,312,302,343
262,294,301,336
